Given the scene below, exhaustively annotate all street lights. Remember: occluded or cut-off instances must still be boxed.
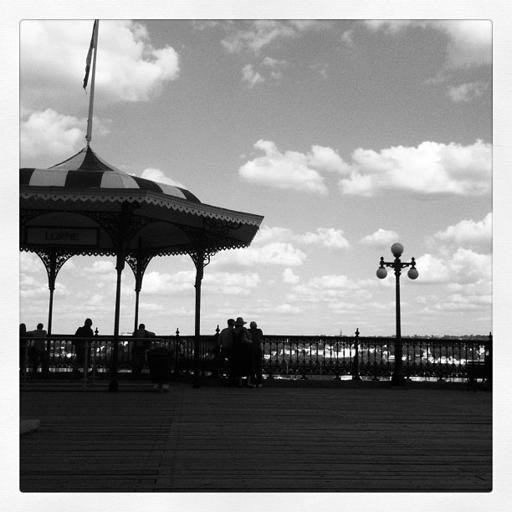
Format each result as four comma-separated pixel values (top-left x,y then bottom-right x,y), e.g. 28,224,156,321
377,243,419,376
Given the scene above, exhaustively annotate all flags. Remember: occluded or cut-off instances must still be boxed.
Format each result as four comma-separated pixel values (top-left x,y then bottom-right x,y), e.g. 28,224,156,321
83,22,95,89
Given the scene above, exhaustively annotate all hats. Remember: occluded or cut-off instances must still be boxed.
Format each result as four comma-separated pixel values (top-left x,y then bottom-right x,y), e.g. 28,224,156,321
235,318,246,324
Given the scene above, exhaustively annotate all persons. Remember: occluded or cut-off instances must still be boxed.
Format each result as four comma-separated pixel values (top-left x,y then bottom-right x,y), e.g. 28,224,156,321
20,318,264,388
